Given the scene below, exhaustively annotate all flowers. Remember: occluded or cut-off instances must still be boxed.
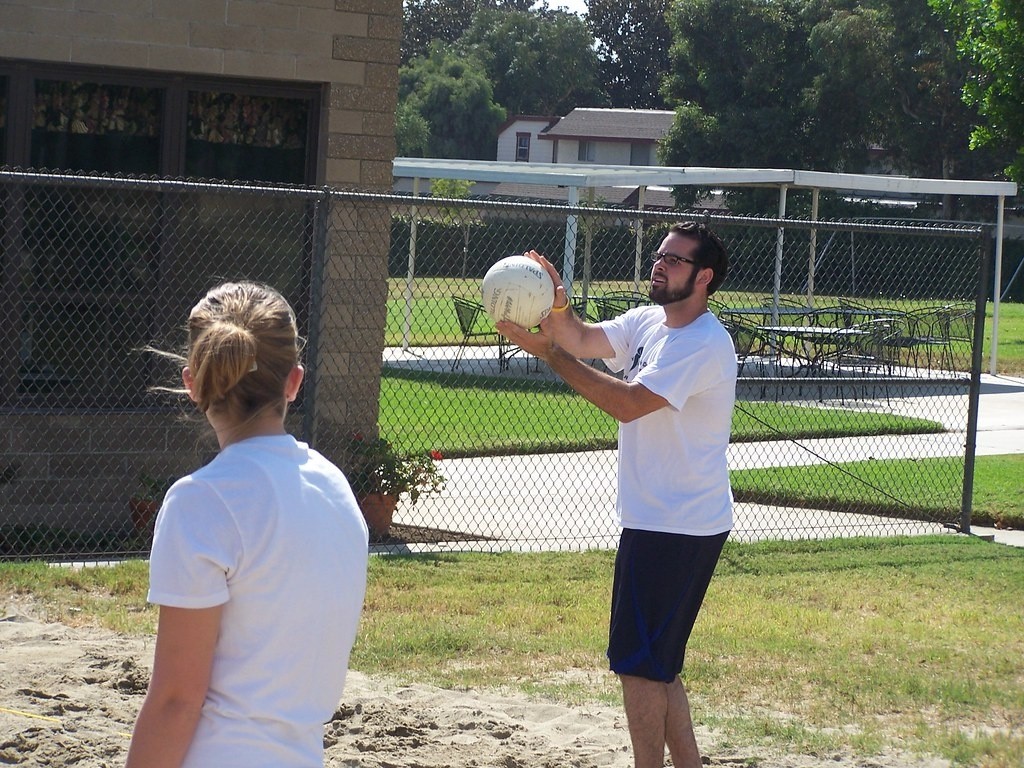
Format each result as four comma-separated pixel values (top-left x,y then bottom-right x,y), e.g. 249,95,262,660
338,433,448,511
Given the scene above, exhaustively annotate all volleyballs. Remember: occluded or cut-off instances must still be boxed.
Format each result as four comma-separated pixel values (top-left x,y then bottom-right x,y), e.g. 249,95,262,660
482,255,555,330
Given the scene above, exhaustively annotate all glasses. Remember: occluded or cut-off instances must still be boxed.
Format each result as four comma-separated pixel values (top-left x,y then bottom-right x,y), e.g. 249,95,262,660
650,252,703,266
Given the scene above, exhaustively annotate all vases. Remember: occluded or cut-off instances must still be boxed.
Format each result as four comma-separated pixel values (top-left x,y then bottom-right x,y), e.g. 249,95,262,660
358,494,398,540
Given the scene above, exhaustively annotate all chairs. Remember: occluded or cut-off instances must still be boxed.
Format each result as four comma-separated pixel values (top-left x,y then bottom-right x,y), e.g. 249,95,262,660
453,290,977,407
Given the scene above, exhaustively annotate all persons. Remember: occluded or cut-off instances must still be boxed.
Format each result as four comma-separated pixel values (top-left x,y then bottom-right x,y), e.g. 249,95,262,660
124,280,370,768
496,222,738,768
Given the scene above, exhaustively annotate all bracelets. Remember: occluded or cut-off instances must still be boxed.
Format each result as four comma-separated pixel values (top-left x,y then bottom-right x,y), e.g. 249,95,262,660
552,296,569,312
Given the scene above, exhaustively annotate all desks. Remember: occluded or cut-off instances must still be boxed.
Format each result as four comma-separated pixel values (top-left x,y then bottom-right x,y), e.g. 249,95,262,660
721,308,827,375
757,325,871,406
818,309,907,377
572,295,647,372
